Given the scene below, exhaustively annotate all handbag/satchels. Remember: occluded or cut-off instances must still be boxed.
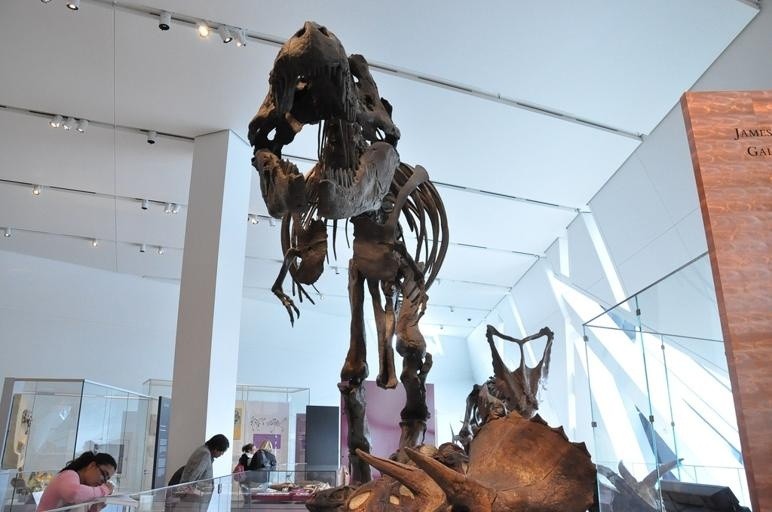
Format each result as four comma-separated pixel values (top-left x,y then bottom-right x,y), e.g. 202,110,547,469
256,449,271,468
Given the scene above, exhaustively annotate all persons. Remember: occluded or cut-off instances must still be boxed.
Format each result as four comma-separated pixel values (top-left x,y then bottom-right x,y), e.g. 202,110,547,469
59,502,106,511
165,466,187,510
248,438,278,487
240,442,255,507
179,435,229,511
36,451,120,511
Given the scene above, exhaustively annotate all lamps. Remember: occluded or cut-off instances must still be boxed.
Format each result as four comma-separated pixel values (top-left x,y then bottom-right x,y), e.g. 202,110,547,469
435,279,441,289
31,184,41,195
163,202,181,215
448,305,456,314
139,198,149,209
156,246,165,257
197,18,211,41
48,113,90,134
249,214,259,224
91,239,100,248
236,27,248,48
66,1,81,10
269,217,277,227
334,266,341,274
145,129,161,145
4,227,14,238
219,24,234,44
140,243,146,253
158,9,172,32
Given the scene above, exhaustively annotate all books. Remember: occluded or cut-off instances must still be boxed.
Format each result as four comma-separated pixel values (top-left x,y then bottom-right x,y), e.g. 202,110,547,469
96,496,139,506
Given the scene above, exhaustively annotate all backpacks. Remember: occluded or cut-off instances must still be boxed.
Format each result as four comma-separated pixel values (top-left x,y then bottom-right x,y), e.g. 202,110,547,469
233,463,246,483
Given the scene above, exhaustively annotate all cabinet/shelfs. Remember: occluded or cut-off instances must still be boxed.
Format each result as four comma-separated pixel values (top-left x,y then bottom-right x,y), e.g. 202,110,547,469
583,249,754,512
45,463,337,512
0,377,160,511
142,379,310,490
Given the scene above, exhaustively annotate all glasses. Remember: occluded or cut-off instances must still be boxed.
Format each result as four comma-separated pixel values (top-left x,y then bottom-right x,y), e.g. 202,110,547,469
95,463,110,484
